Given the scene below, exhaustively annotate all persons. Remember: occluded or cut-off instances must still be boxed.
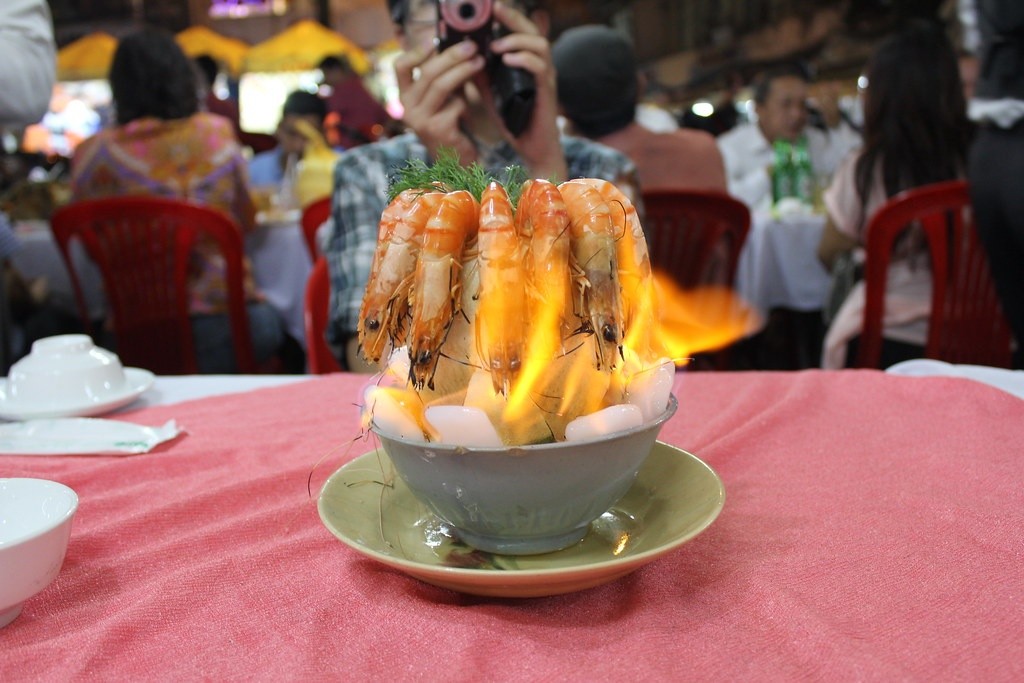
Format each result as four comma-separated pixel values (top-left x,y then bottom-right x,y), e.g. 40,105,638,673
70,27,284,376
0,0,55,129
246,89,345,190
192,56,241,144
315,55,398,150
330,0,634,372
552,25,728,286
816,16,982,367
714,61,855,213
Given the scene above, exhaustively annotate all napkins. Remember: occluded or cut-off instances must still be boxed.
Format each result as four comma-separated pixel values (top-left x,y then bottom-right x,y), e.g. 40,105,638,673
0,416,186,458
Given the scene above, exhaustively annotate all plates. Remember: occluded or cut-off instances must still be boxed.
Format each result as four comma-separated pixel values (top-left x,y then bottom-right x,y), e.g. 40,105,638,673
1,365,157,420
315,439,726,598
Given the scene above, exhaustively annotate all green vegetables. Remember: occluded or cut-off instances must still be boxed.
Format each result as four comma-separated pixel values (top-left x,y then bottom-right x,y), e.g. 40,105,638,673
383,142,564,220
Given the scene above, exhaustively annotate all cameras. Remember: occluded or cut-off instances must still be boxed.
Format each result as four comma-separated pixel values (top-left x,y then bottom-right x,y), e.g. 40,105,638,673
435,0,501,64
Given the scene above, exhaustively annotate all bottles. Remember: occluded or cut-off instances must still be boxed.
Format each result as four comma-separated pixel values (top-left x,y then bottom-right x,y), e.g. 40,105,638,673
769,136,816,206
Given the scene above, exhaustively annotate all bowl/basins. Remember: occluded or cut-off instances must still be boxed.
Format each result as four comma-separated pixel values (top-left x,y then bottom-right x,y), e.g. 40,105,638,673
0,478,80,628
359,393,683,553
6,333,130,406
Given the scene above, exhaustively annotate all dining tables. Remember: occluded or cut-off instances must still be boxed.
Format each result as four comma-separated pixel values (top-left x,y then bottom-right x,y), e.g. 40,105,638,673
12,210,308,355
0,338,1024,683
734,211,835,372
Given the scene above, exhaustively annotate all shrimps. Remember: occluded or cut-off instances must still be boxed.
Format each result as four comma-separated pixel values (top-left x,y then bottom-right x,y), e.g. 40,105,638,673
356,178,652,404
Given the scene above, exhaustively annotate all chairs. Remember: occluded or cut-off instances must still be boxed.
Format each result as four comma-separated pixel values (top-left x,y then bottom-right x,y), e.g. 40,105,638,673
300,193,334,260
857,178,1013,371
303,256,348,375
51,197,255,375
637,189,750,366
